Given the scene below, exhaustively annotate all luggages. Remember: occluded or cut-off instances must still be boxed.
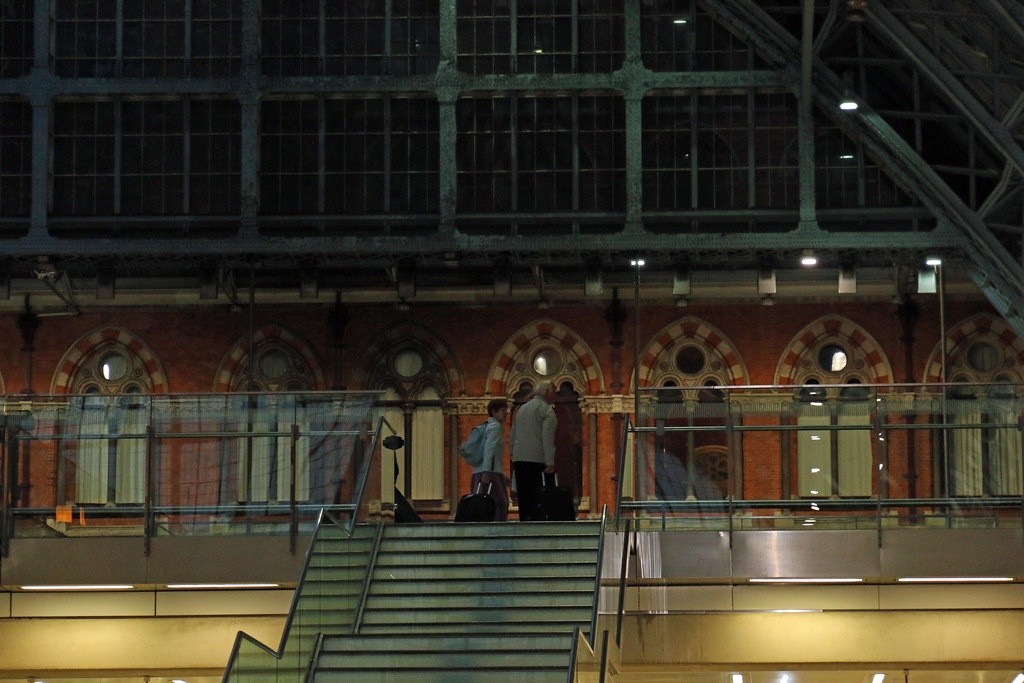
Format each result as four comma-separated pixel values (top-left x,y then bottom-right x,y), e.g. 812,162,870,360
536,470,574,520
456,480,496,522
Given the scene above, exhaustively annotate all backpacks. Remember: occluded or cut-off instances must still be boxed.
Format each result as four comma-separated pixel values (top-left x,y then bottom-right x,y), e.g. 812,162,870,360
462,422,487,466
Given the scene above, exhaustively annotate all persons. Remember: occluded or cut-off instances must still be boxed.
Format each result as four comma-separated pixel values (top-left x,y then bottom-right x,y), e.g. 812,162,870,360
512,382,559,522
470,399,510,521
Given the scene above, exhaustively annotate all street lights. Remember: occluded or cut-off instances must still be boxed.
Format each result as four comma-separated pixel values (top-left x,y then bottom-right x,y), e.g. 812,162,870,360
925,238,954,527
628,249,650,530
